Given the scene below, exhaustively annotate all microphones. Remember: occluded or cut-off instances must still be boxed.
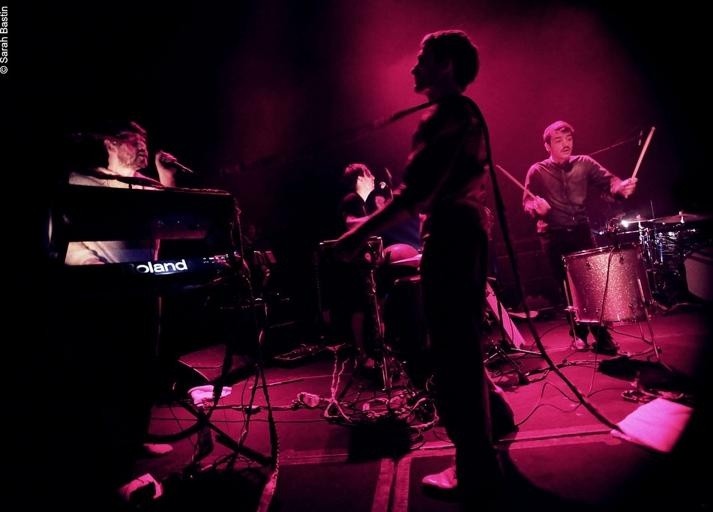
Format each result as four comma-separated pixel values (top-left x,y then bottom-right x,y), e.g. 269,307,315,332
159,156,193,175
638,127,644,147
378,182,394,194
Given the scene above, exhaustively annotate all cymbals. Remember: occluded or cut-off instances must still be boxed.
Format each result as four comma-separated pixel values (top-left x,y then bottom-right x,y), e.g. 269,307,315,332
617,212,704,234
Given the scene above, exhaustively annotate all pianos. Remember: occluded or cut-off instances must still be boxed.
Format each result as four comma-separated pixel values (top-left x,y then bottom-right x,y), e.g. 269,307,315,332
63,247,251,308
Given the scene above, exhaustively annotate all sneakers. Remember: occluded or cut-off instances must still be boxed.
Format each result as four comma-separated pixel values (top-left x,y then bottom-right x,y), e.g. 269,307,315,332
421,448,515,498
574,334,618,354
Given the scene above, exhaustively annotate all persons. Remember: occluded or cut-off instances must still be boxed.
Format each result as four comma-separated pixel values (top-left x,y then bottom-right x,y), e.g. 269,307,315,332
48,114,181,503
519,118,641,356
320,29,523,504
314,161,403,392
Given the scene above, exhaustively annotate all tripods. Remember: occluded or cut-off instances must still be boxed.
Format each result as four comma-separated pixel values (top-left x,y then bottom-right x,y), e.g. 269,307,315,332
561,274,600,364
482,166,545,367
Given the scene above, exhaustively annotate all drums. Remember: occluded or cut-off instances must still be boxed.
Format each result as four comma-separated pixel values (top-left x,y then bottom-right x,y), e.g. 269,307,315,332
683,243,713,301
563,242,655,328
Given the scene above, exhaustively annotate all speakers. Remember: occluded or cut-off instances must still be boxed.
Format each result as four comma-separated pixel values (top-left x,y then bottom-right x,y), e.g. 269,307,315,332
176,342,257,399
